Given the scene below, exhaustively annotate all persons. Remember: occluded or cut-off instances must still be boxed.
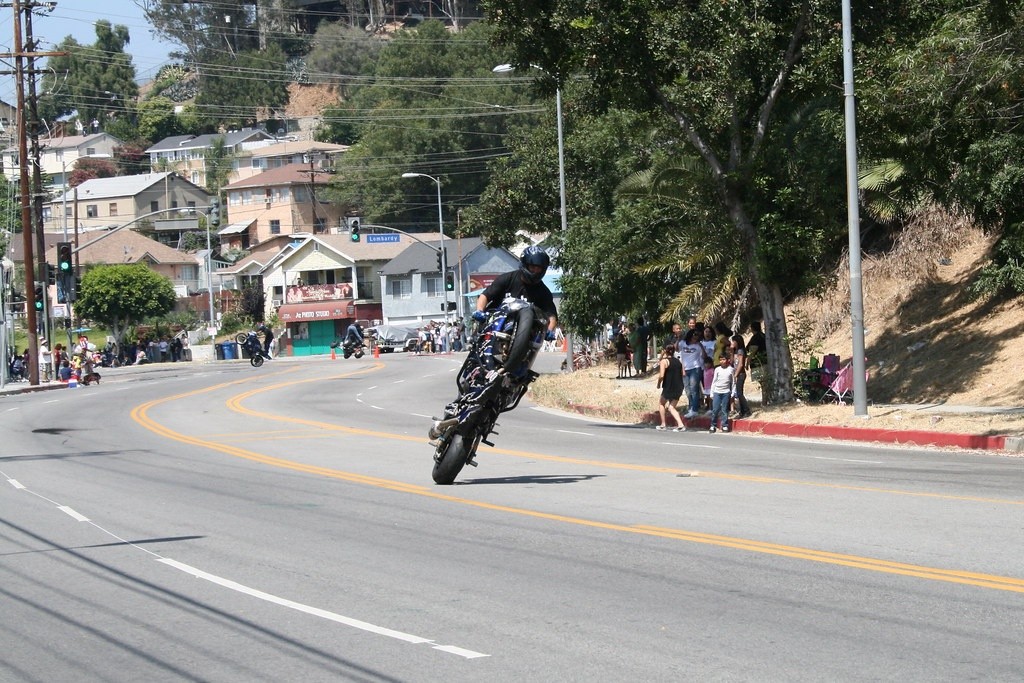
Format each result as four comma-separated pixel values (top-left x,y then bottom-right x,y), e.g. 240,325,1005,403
345,320,367,347
10,333,189,382
412,316,467,355
605,312,766,433
472,245,558,340
297,277,305,287
254,322,273,355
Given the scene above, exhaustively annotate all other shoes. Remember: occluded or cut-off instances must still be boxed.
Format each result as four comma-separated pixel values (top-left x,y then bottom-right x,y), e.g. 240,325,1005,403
733,412,743,421
740,412,751,420
673,425,686,432
656,426,667,430
705,410,712,414
709,426,715,432
42,380,49,383
722,426,729,432
684,410,698,418
728,410,738,419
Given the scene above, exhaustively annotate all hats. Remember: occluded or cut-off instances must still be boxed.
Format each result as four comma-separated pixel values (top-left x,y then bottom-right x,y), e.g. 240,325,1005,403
41,340,47,344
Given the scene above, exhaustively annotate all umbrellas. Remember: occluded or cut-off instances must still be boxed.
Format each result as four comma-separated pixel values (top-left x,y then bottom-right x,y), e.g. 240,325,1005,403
71,327,91,333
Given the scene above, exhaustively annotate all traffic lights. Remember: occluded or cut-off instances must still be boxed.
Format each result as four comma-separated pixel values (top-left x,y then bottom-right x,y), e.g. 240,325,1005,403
436,247,447,273
348,217,361,243
444,271,455,291
35,286,43,311
45,261,56,287
67,275,81,303
57,242,72,274
12,288,21,313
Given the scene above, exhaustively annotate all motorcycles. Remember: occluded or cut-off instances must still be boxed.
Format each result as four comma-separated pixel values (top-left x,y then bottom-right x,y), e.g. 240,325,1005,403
429,296,560,485
331,335,364,360
235,331,272,368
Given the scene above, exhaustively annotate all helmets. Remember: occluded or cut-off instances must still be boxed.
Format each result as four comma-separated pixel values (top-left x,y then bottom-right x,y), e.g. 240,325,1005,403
260,325,265,331
355,319,361,325
521,246,549,283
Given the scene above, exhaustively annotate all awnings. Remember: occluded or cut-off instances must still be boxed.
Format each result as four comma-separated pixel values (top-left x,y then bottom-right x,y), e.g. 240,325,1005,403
279,298,355,322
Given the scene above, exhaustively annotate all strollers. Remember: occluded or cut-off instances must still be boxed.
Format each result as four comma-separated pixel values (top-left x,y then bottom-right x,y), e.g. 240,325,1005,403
815,355,869,406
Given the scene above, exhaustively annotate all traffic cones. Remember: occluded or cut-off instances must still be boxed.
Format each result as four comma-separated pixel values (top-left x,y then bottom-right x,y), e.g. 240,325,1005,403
331,348,335,359
561,337,567,353
373,345,380,358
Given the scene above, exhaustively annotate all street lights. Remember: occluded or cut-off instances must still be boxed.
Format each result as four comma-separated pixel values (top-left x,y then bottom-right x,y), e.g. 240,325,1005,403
402,171,452,353
64,153,113,358
181,209,217,359
492,62,574,375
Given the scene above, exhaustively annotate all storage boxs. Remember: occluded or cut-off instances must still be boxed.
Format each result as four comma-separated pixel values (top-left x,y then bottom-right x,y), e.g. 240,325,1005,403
68,378,78,388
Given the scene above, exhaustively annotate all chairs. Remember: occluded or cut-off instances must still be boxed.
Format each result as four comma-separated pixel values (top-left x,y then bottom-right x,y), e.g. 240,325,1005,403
801,354,841,402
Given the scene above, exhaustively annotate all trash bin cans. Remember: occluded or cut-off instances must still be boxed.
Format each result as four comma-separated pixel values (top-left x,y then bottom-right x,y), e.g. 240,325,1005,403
146,346,162,363
222,342,237,360
216,344,224,359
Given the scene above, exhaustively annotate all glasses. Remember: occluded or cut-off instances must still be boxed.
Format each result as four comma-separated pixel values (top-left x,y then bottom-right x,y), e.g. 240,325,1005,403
693,333,699,336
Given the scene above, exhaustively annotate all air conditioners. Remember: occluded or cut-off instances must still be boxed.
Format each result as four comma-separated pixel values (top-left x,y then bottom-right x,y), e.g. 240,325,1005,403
273,299,283,306
264,198,272,203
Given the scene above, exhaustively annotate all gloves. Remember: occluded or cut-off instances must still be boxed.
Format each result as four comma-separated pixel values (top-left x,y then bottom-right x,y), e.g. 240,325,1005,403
545,331,553,340
472,311,484,322
